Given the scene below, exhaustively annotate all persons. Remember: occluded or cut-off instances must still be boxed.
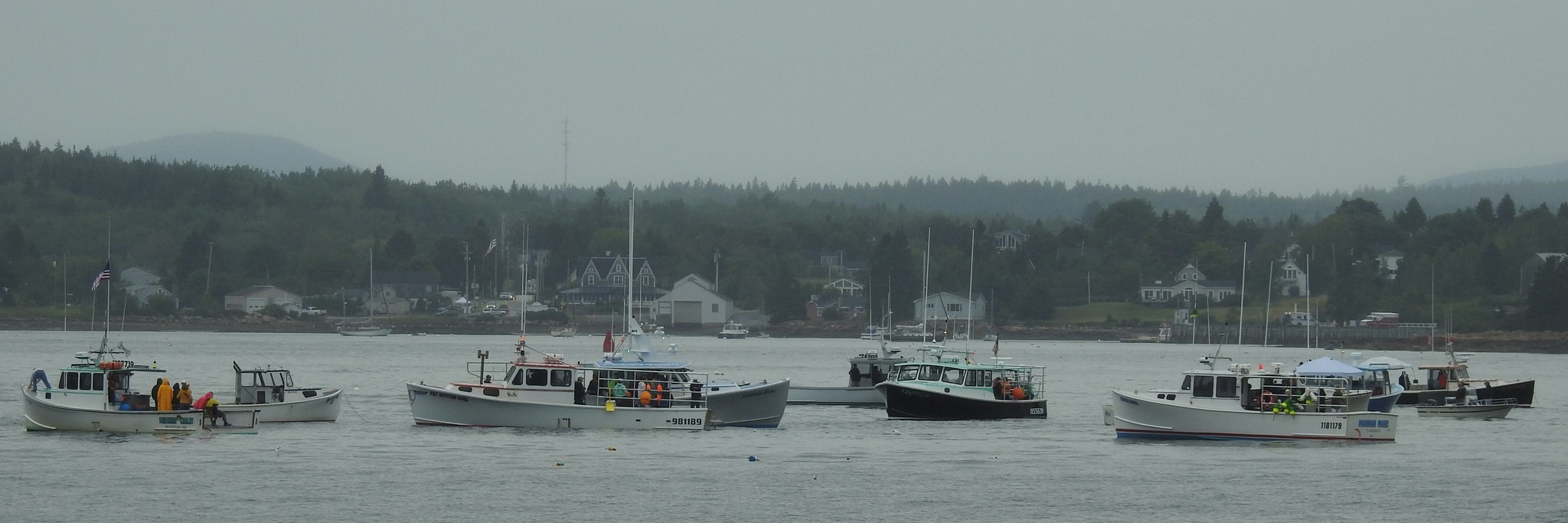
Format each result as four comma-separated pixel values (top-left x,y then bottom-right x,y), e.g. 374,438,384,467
28,368,51,392
120,378,230,427
484,370,703,408
983,376,1011,400
1246,368,1489,413
848,364,940,387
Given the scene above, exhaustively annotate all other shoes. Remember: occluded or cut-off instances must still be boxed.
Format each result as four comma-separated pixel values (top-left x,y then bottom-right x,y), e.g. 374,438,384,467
223,422,232,426
32,389,37,392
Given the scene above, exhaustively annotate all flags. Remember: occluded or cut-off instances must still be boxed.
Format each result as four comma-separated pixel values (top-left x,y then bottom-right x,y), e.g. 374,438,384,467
992,337,999,355
92,262,110,291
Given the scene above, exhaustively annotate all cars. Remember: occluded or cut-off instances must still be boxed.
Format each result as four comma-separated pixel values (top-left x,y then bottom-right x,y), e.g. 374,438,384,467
436,310,459,317
483,295,515,316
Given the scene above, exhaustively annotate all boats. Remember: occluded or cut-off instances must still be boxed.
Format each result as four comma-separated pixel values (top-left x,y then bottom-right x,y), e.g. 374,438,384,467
874,345,1048,421
1103,334,1404,444
1394,342,1535,408
1415,387,1519,419
202,360,343,427
639,322,667,337
20,327,261,435
585,198,790,427
786,339,929,406
717,320,749,339
861,323,945,342
405,335,708,431
982,334,998,341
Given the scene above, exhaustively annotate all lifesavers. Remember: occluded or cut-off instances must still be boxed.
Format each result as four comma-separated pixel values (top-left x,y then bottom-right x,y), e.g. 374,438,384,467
1260,391,1275,409
99,362,123,370
603,353,623,361
542,353,564,362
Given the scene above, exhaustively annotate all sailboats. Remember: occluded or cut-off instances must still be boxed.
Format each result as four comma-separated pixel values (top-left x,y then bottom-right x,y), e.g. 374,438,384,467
336,251,392,336
551,260,576,337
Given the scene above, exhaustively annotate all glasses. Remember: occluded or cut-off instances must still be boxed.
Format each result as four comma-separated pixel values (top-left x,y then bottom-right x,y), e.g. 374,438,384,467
694,380,698,381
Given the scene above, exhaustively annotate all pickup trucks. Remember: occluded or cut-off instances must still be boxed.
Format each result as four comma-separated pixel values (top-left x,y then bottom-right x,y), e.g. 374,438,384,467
303,307,326,315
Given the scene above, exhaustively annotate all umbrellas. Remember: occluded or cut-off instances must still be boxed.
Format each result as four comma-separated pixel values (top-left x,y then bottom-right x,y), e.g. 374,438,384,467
1294,357,1364,377
1357,356,1413,370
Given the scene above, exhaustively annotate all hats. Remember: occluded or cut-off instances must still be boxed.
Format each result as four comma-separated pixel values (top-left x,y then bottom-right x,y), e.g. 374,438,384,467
486,375,492,378
1457,382,1464,386
618,378,622,383
181,380,186,383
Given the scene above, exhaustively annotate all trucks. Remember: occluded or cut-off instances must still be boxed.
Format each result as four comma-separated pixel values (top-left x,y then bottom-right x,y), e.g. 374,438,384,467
1361,312,1399,329
1283,311,1319,327
